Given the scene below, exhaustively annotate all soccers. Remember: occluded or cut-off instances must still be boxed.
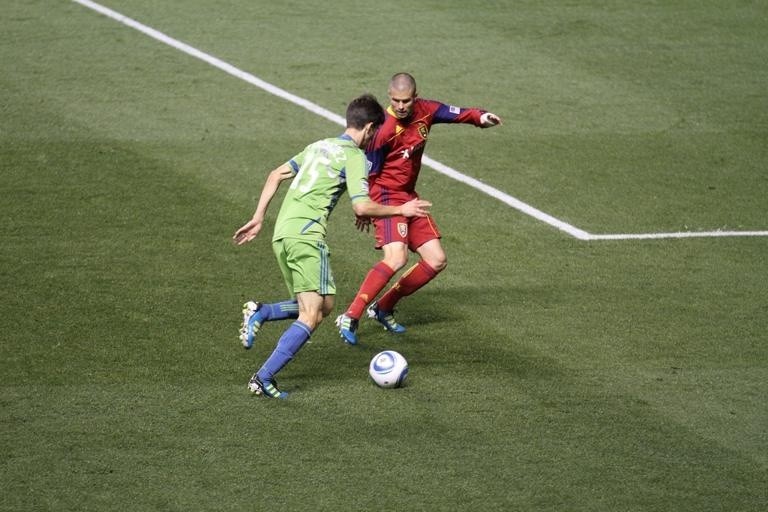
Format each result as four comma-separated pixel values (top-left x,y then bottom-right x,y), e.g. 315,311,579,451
368,350,409,388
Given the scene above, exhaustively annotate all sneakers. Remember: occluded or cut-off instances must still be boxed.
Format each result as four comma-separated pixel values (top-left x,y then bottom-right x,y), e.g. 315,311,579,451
367,301,406,334
335,313,359,345
248,371,287,398
238,300,266,349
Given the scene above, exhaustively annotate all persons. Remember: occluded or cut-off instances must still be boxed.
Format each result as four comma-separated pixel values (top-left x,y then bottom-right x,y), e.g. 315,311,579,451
334,74,504,346
233,94,433,399
